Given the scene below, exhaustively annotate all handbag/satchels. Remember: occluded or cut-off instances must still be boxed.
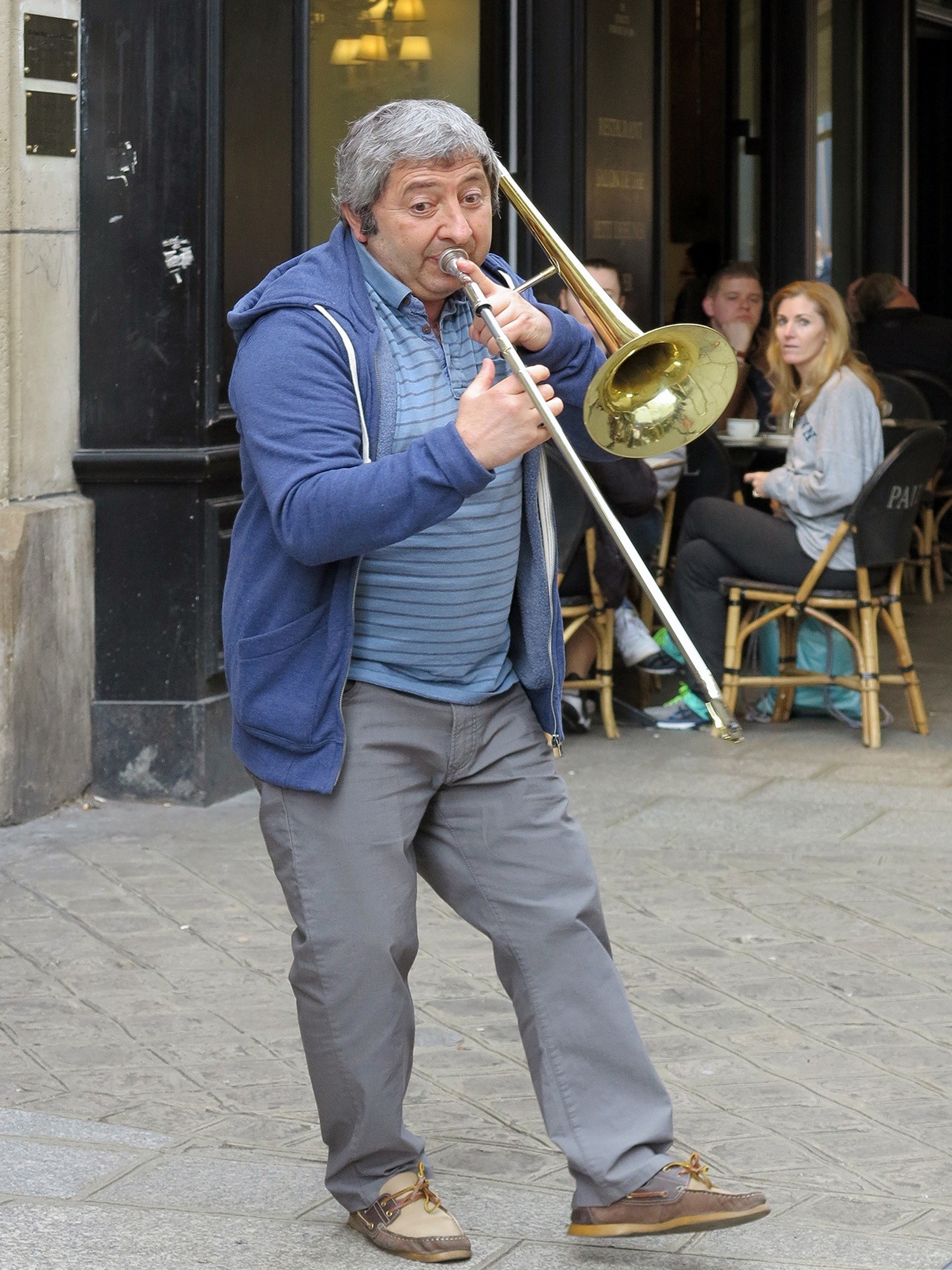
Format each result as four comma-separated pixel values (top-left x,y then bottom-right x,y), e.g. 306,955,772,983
741,599,893,726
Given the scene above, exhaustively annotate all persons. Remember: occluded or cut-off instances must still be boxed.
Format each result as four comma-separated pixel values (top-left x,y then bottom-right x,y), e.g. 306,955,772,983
559,257,686,733
223,100,769,1265
669,243,731,329
845,274,952,579
702,262,785,516
643,282,884,727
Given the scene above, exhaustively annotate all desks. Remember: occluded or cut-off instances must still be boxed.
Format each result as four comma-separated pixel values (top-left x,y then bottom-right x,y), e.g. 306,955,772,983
634,430,788,637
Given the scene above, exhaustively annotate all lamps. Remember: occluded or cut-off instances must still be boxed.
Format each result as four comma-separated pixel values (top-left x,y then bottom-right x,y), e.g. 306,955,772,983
327,0,431,68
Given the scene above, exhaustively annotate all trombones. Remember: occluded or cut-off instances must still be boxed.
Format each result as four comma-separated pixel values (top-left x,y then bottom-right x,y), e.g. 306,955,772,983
436,138,744,744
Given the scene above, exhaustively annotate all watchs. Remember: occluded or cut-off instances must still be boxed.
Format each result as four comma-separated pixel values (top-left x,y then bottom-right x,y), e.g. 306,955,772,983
732,347,747,359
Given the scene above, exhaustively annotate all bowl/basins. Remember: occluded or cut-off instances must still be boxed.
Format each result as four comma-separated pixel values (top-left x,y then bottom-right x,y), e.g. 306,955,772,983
726,419,760,437
761,434,791,447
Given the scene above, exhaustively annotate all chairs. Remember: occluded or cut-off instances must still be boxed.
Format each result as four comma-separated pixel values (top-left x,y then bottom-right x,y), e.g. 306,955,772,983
548,369,952,750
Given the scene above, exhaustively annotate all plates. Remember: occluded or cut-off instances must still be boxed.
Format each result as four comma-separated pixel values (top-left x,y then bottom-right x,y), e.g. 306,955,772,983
716,435,762,446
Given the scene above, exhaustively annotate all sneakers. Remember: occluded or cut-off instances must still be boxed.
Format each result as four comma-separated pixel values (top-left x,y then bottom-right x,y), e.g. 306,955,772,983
558,695,594,732
610,597,659,665
640,679,712,730
633,649,680,674
566,1151,771,1237
347,1163,472,1262
